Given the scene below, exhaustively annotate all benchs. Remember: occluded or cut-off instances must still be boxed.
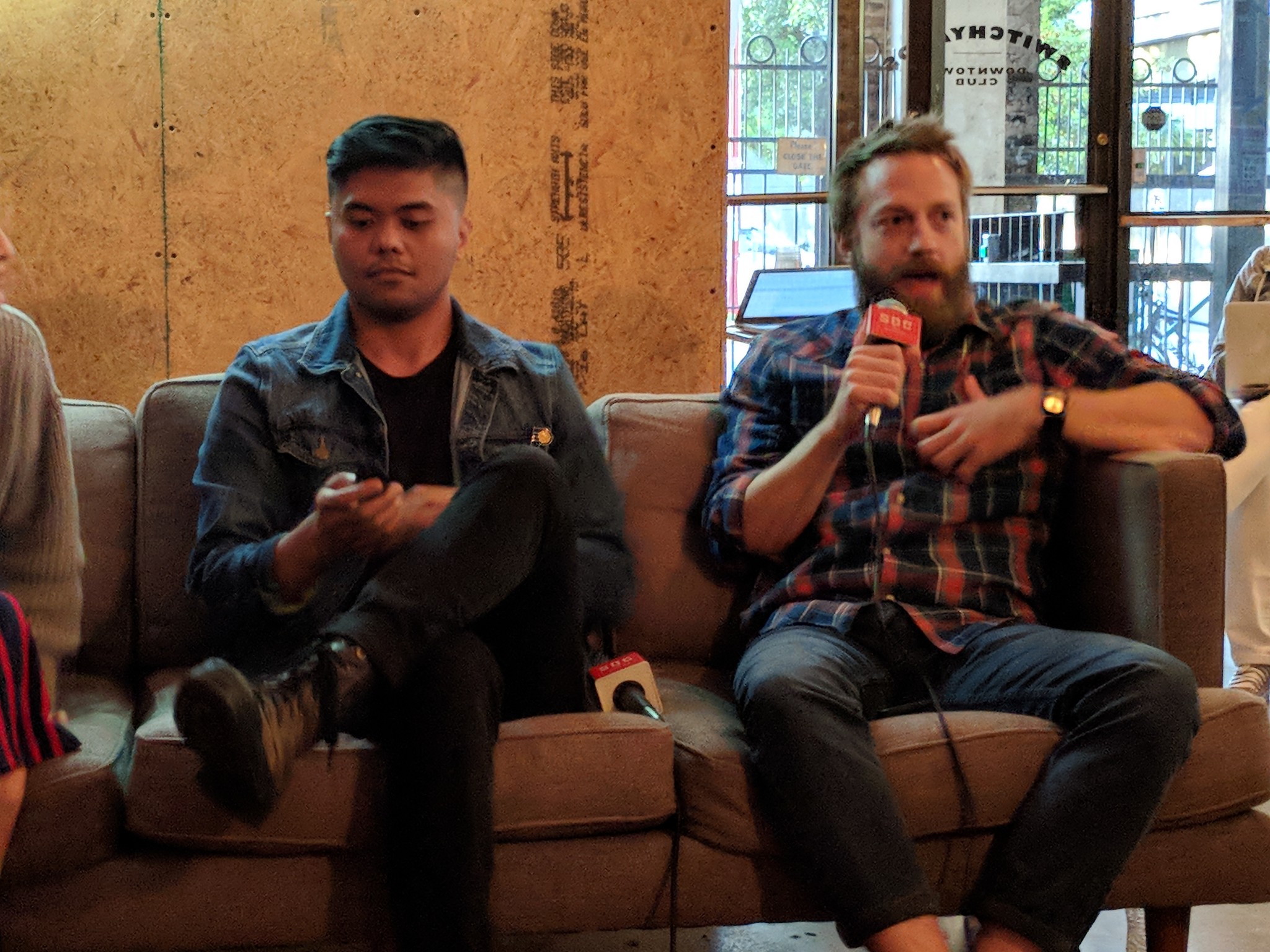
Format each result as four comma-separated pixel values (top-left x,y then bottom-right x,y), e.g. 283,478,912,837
0,373,1270,952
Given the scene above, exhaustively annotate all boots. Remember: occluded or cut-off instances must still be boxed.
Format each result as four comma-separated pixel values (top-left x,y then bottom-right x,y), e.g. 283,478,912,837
175,635,365,832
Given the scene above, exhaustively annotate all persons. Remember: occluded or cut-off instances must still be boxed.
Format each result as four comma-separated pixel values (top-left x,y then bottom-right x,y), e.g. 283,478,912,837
684,109,1249,952
1209,245,1270,709
172,114,633,952
1004,114,1038,177
0,227,91,857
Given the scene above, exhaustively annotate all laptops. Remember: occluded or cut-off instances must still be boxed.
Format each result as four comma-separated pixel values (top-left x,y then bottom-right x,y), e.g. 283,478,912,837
1224,301,1269,398
736,265,862,334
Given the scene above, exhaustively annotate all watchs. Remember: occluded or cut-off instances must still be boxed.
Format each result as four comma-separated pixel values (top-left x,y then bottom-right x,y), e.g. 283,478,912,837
1038,382,1069,450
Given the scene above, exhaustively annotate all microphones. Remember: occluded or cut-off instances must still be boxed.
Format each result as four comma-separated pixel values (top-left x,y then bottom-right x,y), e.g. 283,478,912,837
852,298,922,435
587,650,664,723
